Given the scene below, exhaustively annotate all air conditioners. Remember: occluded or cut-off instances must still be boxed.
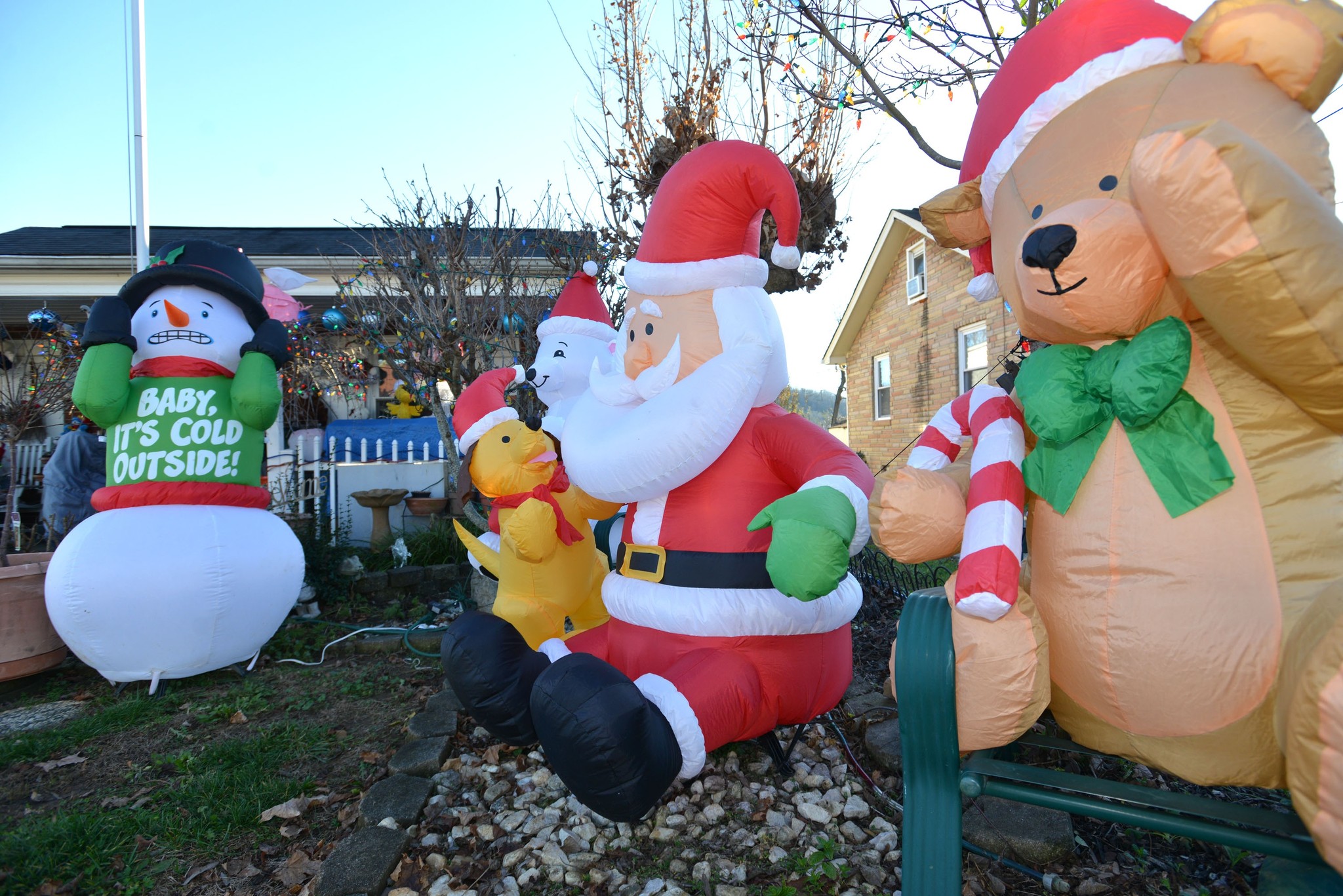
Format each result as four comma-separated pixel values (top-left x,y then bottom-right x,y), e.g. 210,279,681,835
907,275,924,298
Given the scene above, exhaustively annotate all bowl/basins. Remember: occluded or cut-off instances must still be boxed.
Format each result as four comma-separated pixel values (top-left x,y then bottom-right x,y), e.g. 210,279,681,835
403,497,448,516
411,491,431,498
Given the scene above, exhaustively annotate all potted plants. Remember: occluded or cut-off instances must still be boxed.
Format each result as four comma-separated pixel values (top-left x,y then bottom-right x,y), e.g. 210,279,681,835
0,312,95,683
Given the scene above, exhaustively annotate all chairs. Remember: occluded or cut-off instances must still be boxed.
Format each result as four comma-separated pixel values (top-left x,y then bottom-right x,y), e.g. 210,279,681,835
895,586,1343,896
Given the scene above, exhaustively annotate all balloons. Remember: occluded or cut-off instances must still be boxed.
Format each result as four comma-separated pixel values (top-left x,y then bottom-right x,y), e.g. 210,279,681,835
503,312,525,333
361,310,382,328
870,0,1343,876
45,239,307,696
296,303,312,329
539,309,551,324
27,310,59,330
441,141,874,824
322,306,347,330
